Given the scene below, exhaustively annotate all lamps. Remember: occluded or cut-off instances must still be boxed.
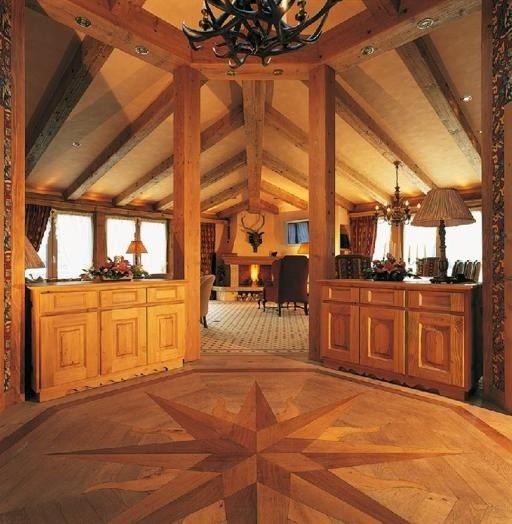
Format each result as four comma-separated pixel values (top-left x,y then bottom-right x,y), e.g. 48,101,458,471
410,187,476,284
182,0,341,67
23,236,46,268
375,160,421,227
126,241,147,279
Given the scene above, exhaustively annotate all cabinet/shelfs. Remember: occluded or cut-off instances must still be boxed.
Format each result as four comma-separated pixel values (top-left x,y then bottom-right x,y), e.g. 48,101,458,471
26,279,187,402
317,280,484,400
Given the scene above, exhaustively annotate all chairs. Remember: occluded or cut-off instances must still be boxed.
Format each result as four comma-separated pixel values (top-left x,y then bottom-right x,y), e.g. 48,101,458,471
416,256,449,277
200,275,216,327
336,255,364,279
258,254,309,317
450,260,481,284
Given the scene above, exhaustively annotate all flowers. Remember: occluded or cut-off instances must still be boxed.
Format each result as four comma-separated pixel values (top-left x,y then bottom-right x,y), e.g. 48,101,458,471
80,255,149,280
362,253,407,281
